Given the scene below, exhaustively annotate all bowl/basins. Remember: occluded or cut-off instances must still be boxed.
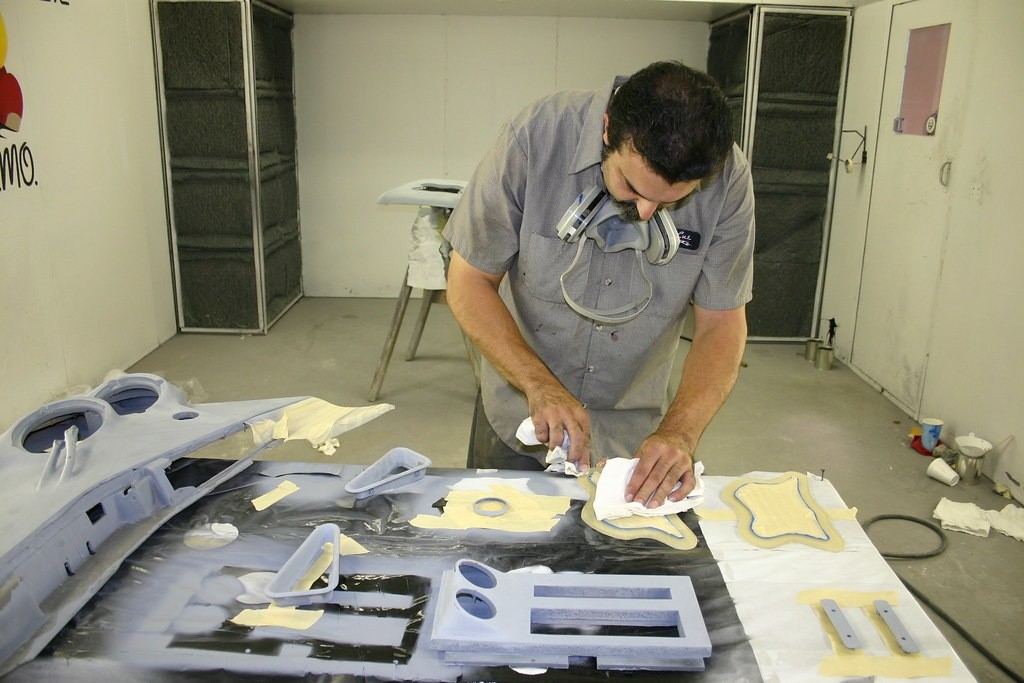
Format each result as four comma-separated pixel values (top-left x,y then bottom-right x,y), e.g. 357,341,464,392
955,432,992,458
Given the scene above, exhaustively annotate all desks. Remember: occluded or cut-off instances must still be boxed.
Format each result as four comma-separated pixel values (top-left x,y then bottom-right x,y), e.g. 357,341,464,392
0,458,978,683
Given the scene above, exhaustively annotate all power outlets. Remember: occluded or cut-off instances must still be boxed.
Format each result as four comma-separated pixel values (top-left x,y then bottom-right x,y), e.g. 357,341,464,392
969,182,983,201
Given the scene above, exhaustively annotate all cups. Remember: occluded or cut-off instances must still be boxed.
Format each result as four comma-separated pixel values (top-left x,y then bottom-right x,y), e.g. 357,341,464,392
920,418,944,453
926,457,960,486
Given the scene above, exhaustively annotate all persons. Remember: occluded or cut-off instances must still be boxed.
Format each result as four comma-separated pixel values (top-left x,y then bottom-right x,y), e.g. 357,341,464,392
440,59,756,509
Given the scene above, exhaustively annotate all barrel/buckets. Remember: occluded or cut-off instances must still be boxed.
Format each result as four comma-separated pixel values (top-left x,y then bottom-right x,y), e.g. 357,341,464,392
957,450,985,482
805,339,824,362
814,347,834,370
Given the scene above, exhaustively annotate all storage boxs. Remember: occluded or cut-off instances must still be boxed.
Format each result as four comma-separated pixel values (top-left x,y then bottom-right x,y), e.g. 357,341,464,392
172,159,299,249
721,96,845,182
745,264,827,342
746,183,836,264
178,231,304,335
157,0,294,90
165,89,295,171
706,5,855,103
